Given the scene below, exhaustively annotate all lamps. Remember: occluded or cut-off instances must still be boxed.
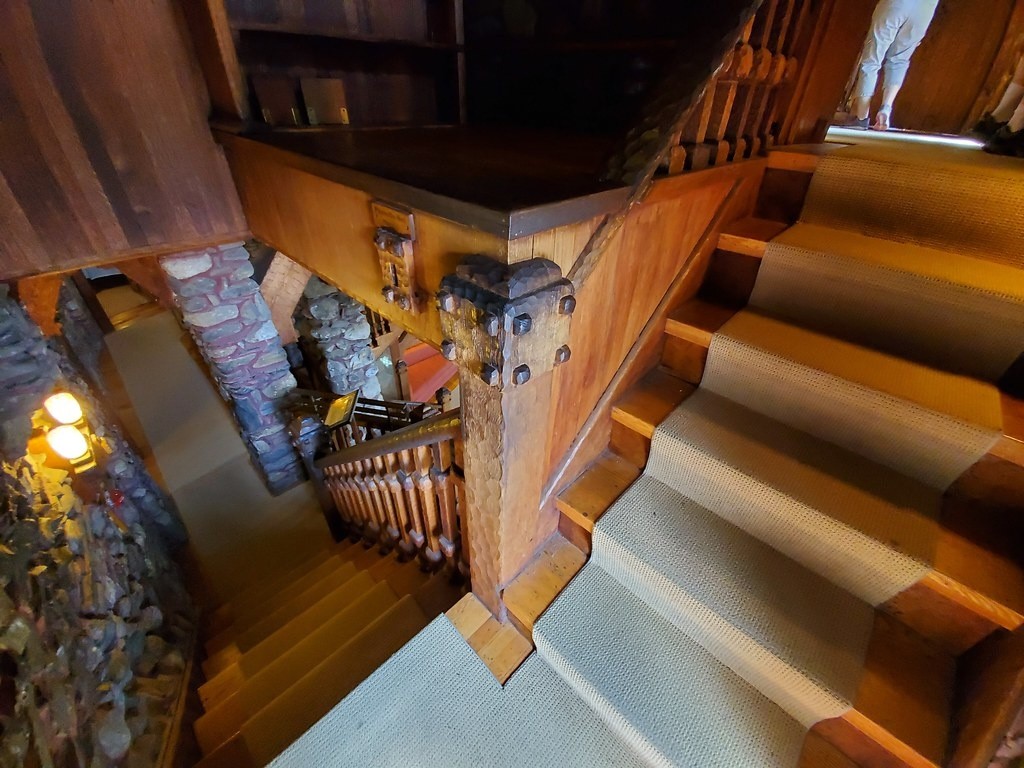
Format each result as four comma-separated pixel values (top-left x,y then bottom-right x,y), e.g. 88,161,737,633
44,392,82,423
46,425,89,459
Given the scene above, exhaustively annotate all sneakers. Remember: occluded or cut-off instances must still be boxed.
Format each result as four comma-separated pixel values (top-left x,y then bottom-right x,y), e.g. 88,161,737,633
839,115,871,131
982,125,1024,158
872,105,889,132
969,111,1009,144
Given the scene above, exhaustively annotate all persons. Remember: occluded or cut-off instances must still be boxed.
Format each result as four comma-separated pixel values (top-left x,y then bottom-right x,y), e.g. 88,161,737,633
969,54,1024,160
830,0,940,132
